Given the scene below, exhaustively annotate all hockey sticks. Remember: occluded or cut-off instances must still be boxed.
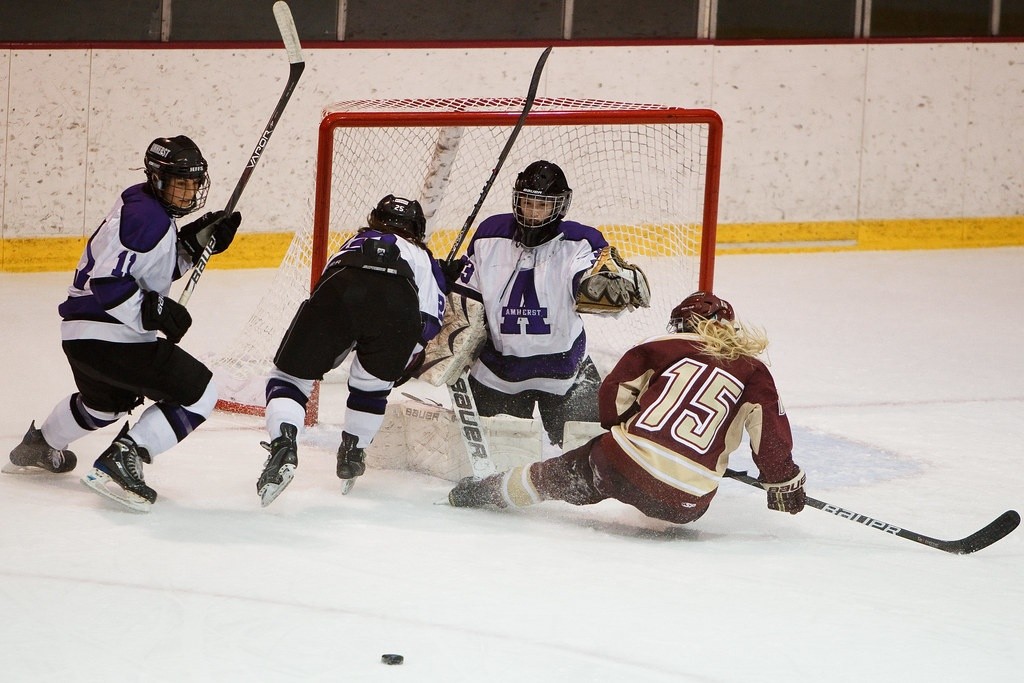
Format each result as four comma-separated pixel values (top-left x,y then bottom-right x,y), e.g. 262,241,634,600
446,369,498,483
725,467,1021,555
178,0,309,307
446,42,555,265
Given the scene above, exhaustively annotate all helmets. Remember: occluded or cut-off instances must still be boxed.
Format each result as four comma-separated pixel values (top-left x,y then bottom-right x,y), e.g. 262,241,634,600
512,160,572,228
370,194,426,238
144,135,211,218
666,291,735,333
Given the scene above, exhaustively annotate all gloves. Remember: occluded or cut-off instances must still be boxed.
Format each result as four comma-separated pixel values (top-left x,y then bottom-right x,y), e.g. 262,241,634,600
767,487,807,515
142,290,192,345
180,211,242,266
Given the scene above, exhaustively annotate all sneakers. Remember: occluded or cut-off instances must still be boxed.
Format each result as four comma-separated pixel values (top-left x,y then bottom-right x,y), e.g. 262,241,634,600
256,422,298,495
10,419,78,473
449,475,472,509
93,421,157,504
336,431,365,479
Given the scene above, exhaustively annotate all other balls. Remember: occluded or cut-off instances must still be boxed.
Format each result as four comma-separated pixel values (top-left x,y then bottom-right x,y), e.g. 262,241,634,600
382,654,404,665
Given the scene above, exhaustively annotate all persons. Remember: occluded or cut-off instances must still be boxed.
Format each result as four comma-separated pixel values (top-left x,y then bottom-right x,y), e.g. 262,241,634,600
10,135,242,504
256,194,464,499
449,292,806,524
455,160,651,449
